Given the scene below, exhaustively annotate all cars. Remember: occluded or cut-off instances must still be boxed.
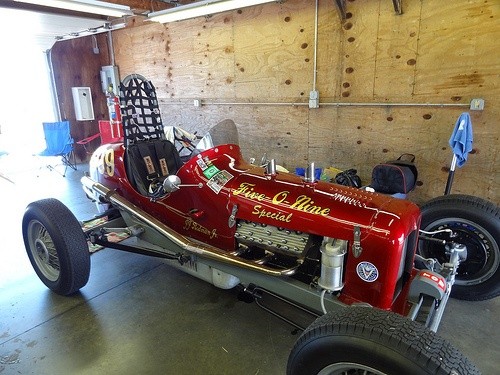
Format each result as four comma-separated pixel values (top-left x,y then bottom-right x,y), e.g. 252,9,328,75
21,72,500,375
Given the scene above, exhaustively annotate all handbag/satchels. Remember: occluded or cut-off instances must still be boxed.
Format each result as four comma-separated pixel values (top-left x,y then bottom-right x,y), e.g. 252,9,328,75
372,152,420,197
295,165,322,181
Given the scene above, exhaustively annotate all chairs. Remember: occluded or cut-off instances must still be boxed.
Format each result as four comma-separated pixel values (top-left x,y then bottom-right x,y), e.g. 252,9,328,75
37,120,77,177
76,120,124,156
123,138,182,198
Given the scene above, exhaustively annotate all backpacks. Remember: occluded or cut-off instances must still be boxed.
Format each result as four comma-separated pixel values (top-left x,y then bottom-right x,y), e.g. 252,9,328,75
334,168,362,189
